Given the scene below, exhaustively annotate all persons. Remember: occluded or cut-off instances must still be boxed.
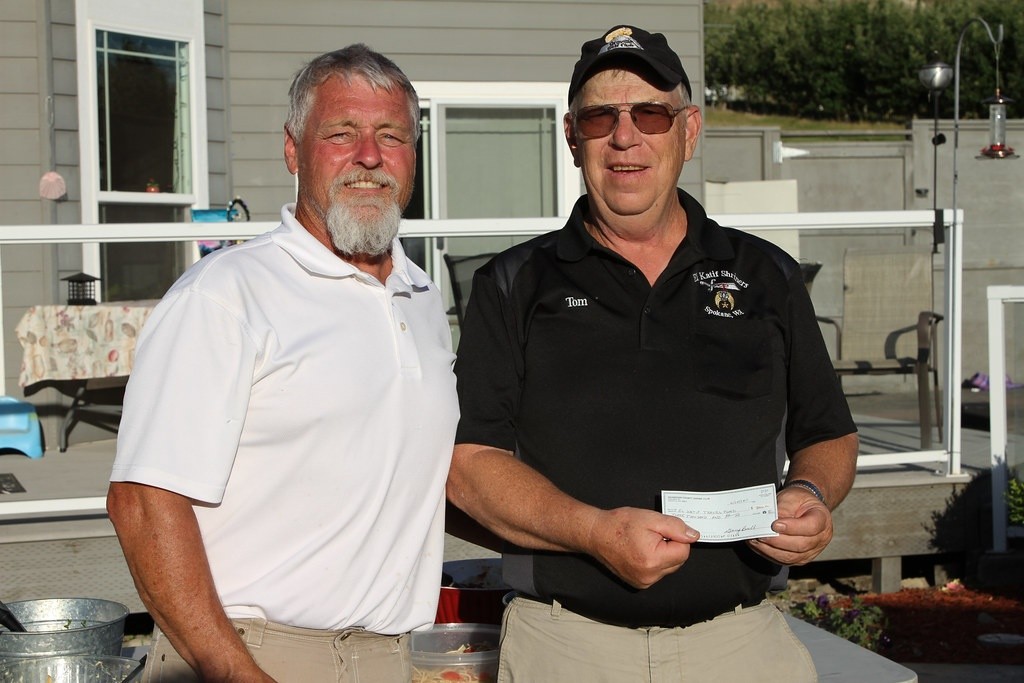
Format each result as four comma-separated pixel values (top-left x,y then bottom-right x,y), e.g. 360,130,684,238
106,42,461,683
445,25,859,683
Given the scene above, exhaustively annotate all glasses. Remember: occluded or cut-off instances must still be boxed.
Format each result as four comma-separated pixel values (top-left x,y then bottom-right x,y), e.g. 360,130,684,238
574,101,689,137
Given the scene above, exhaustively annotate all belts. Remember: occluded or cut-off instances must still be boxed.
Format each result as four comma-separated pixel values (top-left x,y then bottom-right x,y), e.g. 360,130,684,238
519,592,768,628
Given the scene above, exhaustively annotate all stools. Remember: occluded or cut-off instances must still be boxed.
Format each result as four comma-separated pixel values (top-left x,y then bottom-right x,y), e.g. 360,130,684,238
0,396,44,460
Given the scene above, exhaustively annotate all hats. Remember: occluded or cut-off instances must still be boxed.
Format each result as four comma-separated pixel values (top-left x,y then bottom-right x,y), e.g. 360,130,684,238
567,25,693,105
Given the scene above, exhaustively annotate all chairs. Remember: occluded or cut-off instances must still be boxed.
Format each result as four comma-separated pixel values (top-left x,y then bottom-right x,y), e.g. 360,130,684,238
816,311,944,450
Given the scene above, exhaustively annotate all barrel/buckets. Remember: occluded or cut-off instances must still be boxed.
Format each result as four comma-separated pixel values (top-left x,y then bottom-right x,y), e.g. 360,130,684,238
433,557,513,624
0,598,154,683
410,623,501,683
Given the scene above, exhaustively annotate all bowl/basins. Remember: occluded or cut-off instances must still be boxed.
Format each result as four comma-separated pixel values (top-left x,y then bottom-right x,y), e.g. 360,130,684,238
0,655,144,683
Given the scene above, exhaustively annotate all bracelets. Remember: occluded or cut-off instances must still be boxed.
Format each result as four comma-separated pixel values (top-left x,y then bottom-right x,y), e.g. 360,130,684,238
779,479,827,506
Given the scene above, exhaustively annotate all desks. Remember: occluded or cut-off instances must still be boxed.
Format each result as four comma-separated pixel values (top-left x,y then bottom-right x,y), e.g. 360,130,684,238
15,298,161,452
783,614,919,683
